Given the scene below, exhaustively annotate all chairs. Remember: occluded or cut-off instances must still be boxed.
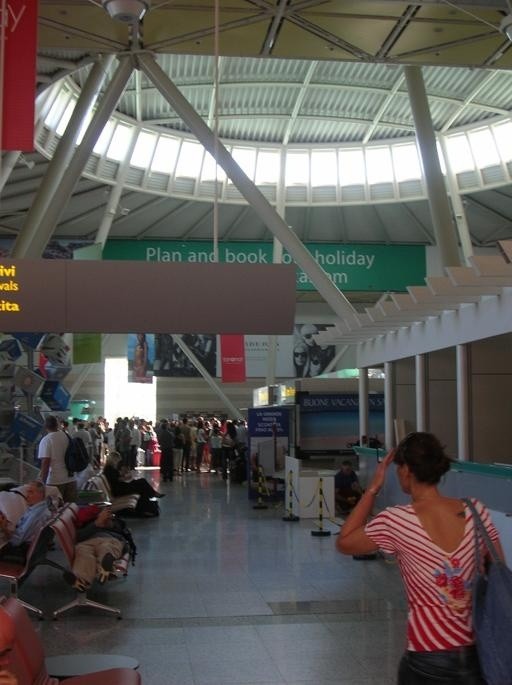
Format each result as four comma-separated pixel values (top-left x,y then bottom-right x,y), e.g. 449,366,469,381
1,465,146,684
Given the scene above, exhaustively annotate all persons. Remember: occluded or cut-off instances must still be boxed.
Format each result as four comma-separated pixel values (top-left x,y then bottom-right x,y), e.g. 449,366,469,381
134,333,148,378
36,416,81,500
292,325,333,375
64,505,128,590
103,451,166,502
336,462,361,509
335,431,501,683
11,481,47,545
59,413,249,476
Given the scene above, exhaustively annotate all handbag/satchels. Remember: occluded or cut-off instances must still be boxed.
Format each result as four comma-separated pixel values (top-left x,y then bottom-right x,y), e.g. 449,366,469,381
472,560,512,684
64,438,89,471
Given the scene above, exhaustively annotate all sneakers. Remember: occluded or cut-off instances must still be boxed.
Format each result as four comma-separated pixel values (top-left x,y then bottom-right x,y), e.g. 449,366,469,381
64,571,88,593
95,552,114,585
